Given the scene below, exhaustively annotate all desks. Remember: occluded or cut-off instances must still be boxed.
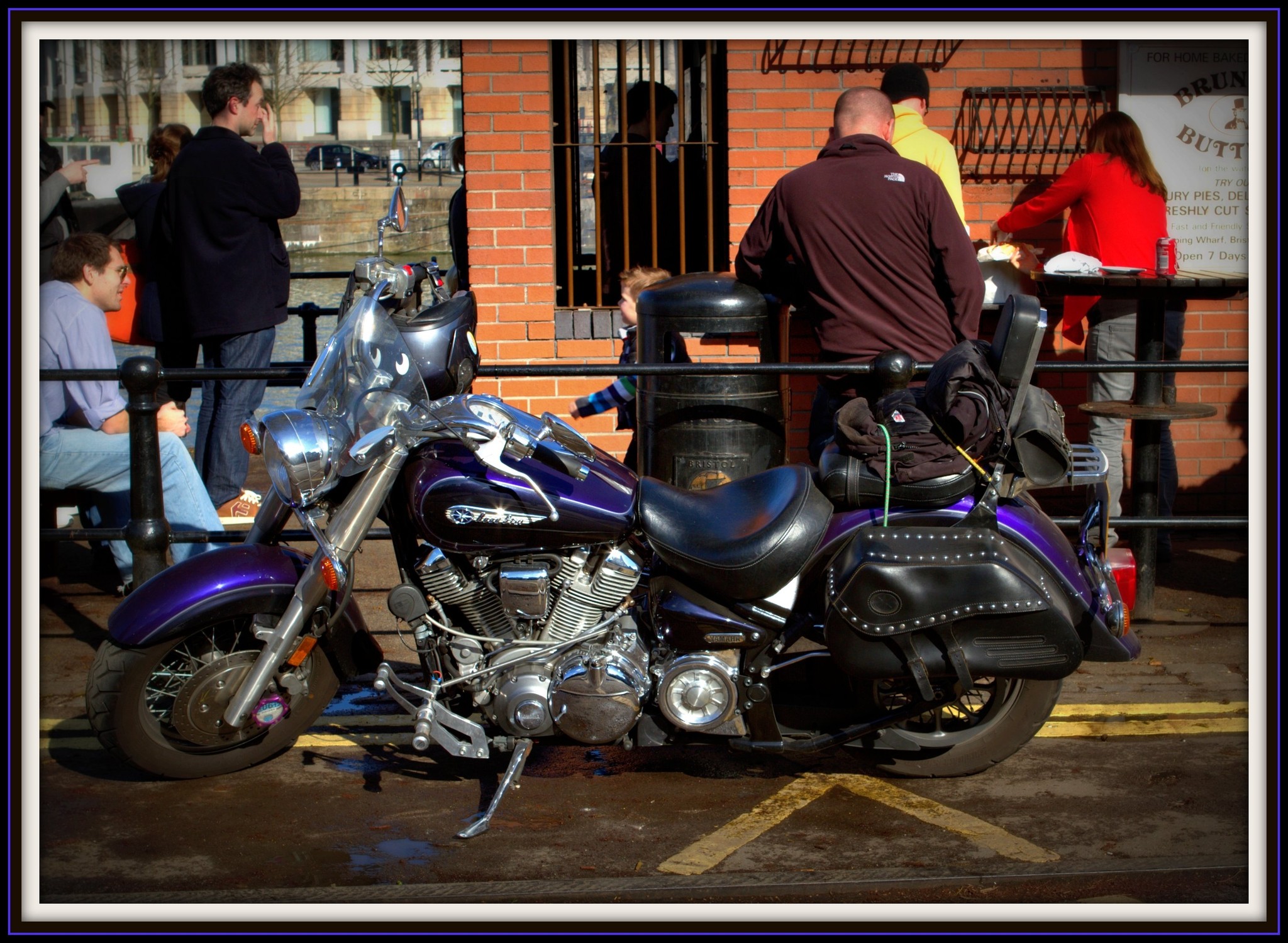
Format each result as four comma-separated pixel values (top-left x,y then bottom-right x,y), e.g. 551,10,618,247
1032,266,1249,623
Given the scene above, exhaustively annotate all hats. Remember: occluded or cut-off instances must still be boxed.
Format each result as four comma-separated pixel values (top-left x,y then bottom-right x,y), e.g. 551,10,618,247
880,62,931,107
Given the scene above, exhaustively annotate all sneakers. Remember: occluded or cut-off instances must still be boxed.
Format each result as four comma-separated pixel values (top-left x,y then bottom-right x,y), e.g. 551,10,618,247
216,486,265,524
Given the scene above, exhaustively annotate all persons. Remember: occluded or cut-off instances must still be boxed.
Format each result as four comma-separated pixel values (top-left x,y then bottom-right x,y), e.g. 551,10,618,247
988,110,1180,553
590,80,698,308
39,231,233,596
566,265,693,478
164,60,301,514
738,61,987,476
39,120,199,434
446,130,470,306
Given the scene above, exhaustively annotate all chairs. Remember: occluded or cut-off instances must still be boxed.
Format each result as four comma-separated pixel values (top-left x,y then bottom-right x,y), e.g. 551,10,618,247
821,292,1046,508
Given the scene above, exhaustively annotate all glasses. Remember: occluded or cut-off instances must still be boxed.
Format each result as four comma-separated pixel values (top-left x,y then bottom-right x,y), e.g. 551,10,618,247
113,264,128,280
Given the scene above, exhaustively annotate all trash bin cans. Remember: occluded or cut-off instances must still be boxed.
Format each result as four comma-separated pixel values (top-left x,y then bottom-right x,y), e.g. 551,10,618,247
636,271,786,492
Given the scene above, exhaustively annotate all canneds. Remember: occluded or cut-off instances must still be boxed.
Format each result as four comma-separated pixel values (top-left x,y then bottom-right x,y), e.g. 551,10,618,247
1155,237,1179,276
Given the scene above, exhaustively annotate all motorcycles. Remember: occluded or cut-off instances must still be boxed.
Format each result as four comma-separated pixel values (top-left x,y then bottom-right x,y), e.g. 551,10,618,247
100,166,1140,840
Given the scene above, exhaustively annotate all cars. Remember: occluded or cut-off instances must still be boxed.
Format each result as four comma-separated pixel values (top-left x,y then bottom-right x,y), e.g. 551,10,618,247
305,145,379,171
418,141,451,168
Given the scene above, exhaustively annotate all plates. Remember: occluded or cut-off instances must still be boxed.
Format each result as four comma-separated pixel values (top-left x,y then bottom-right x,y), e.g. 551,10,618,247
1098,266,1147,274
